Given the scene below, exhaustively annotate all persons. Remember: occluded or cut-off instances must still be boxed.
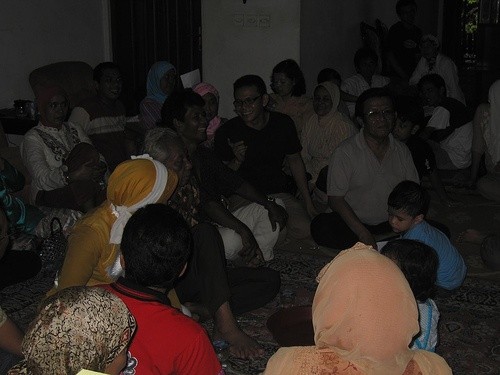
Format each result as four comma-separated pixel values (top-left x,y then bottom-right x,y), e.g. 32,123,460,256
387,180,468,295
7,285,138,375
284,34,500,214
310,88,451,251
381,240,440,353
88,204,226,375
262,241,453,375
381,0,425,105
0,58,319,359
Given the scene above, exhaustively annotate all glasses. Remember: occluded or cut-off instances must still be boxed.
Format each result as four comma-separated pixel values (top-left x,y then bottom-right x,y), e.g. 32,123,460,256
363,107,397,122
233,94,262,107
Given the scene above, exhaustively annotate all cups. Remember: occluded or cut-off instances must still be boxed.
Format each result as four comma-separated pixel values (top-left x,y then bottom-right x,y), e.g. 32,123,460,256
13,99,26,118
212,340,232,370
281,288,296,310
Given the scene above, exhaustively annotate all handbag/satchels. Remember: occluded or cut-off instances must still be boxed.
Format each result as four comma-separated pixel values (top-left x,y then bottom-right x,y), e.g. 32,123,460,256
36,217,69,277
13,100,38,121
